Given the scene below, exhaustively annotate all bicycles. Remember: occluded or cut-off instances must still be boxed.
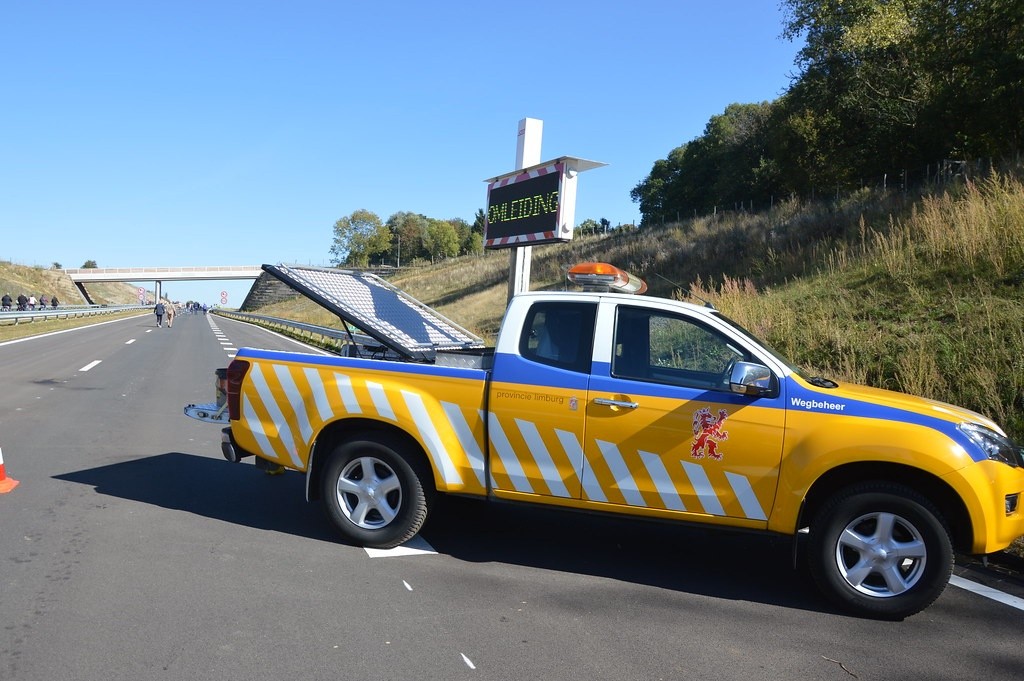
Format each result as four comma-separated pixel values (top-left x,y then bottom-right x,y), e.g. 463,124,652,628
1,300,59,312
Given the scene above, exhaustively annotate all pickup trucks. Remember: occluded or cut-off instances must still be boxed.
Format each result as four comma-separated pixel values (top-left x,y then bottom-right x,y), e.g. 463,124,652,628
183,262,1024,622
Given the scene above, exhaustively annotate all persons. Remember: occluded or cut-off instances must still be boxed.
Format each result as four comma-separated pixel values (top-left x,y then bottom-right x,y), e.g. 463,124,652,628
2,292,59,311
142,299,207,329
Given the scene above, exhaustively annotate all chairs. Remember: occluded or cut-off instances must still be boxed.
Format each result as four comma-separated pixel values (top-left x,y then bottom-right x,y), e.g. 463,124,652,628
537,309,581,365
